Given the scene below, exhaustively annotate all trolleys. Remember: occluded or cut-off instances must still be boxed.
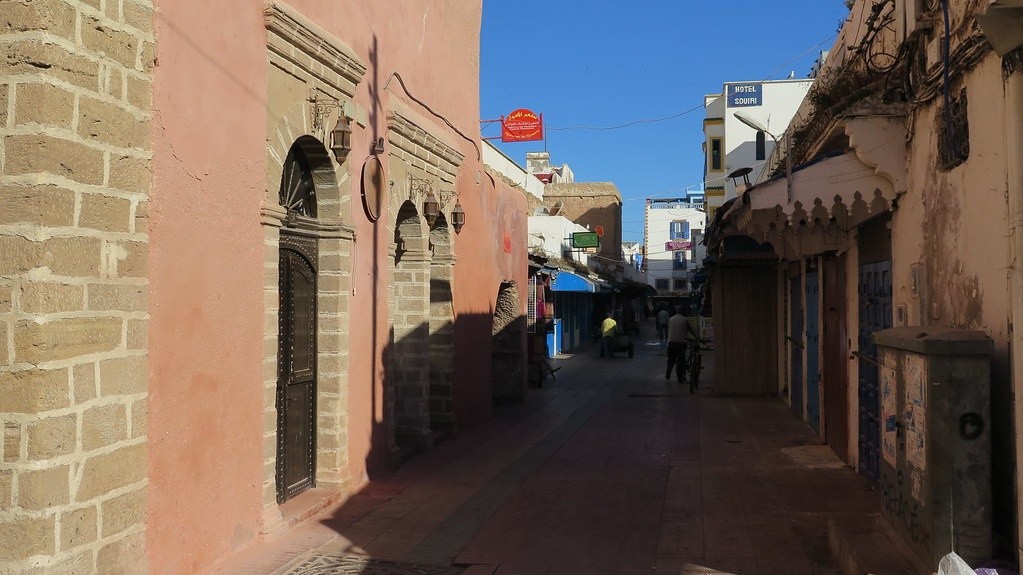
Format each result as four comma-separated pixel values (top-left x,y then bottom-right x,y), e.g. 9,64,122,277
599,333,634,360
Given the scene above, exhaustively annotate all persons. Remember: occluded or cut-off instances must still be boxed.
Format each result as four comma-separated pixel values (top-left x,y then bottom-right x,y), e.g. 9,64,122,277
600,313,620,359
657,307,670,338
664,305,701,384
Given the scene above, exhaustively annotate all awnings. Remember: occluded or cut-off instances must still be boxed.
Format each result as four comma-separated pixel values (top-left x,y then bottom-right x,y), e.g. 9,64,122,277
549,272,600,293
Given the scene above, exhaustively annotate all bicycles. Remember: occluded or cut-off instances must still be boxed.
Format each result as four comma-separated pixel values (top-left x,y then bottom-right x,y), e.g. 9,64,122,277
674,337,715,394
